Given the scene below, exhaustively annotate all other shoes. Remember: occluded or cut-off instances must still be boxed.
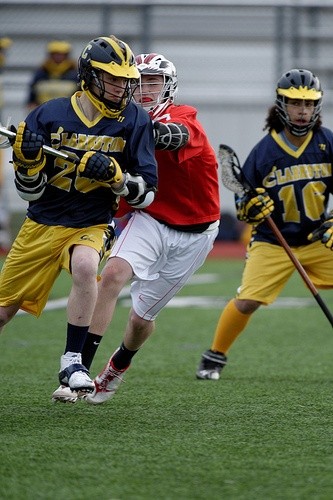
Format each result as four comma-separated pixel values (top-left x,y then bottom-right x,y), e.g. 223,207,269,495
195,349,228,380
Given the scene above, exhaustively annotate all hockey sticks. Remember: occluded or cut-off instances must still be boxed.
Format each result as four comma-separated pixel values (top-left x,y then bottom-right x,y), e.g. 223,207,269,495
1,128,86,168
218,143,333,324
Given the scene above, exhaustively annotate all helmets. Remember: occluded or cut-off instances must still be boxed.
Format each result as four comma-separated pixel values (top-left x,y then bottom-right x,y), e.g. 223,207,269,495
275,69,323,136
78,34,142,119
47,40,72,52
130,53,179,113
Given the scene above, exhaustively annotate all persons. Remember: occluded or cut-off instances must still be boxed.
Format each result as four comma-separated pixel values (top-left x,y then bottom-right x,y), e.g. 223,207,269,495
196,68,333,381
53,53,221,405
23,40,82,116
0,35,159,394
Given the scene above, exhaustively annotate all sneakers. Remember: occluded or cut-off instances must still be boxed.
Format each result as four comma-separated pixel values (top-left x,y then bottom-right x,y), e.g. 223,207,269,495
51,384,78,404
82,347,132,405
59,351,96,394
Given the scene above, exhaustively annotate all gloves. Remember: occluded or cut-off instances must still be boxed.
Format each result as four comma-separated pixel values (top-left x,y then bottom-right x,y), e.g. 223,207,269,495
307,216,333,252
237,187,274,226
76,151,122,187
8,120,46,175
